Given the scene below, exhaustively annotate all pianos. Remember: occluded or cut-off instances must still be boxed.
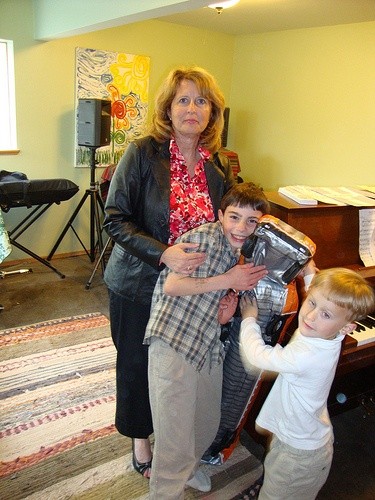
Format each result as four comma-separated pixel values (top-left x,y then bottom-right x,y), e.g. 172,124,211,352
242,188,375,451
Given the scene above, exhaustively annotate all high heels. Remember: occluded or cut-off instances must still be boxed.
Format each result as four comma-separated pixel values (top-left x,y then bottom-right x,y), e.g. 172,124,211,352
131,438,153,480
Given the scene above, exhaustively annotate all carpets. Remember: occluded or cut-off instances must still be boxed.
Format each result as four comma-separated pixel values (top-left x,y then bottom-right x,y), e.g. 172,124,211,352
0,310,266,500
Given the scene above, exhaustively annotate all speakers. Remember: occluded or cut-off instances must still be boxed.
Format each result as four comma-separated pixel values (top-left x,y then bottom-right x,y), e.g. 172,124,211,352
78,98,112,146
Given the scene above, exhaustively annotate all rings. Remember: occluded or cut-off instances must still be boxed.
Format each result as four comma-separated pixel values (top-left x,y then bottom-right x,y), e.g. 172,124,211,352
188,265,192,271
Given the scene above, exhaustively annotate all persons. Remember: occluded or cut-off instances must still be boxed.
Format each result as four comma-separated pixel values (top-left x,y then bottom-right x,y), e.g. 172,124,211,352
103,68,236,478
239,260,374,500
142,181,270,500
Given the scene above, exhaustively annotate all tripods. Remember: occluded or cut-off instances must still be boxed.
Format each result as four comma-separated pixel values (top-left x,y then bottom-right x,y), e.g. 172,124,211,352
47,146,104,277
